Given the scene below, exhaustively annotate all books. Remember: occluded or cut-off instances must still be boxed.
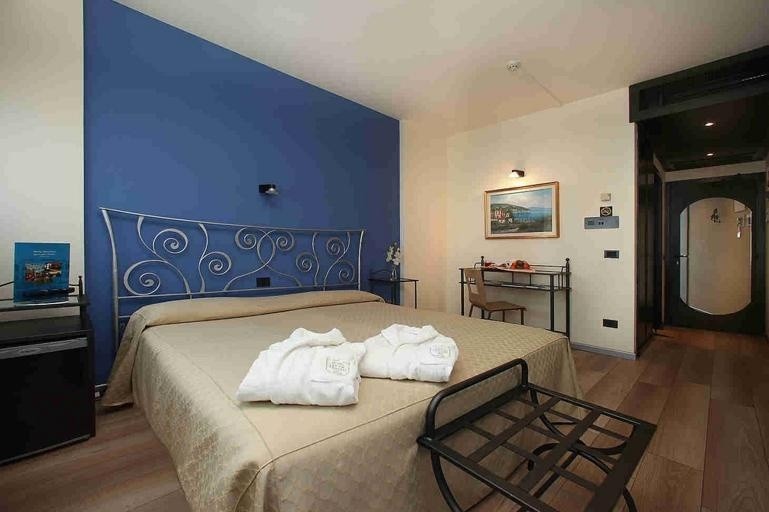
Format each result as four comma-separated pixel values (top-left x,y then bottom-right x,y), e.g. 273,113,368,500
12,240,71,303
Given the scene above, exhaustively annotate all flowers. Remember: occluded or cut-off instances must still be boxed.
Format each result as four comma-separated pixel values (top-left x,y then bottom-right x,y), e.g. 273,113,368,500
384,240,401,266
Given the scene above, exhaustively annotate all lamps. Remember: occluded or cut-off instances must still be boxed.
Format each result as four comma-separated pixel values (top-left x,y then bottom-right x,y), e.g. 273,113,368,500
260,184,278,195
510,169,524,177
710,208,721,223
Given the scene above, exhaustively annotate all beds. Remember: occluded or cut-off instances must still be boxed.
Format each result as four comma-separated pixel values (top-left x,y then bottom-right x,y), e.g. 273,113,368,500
101,204,581,512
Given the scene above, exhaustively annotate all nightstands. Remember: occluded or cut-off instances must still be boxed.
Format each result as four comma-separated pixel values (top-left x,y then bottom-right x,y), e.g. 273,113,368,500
0,276,95,465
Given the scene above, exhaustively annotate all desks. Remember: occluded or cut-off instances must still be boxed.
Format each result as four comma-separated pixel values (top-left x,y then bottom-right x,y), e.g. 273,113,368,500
459,256,572,341
368,278,419,309
417,358,658,512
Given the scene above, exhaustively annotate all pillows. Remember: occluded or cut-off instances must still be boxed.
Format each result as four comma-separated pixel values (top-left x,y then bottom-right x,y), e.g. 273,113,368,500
141,287,380,327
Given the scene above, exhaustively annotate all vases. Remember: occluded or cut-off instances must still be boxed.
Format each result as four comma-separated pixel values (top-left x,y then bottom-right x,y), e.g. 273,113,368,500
390,263,398,279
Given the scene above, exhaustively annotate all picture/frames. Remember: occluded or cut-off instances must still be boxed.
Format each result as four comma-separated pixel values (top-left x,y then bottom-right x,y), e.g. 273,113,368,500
483,181,560,240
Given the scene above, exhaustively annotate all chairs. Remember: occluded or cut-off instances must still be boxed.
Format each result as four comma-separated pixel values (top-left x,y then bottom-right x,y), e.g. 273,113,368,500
465,269,527,325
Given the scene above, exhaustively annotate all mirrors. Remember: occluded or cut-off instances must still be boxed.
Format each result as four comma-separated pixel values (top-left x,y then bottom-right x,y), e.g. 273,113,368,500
677,194,755,318
